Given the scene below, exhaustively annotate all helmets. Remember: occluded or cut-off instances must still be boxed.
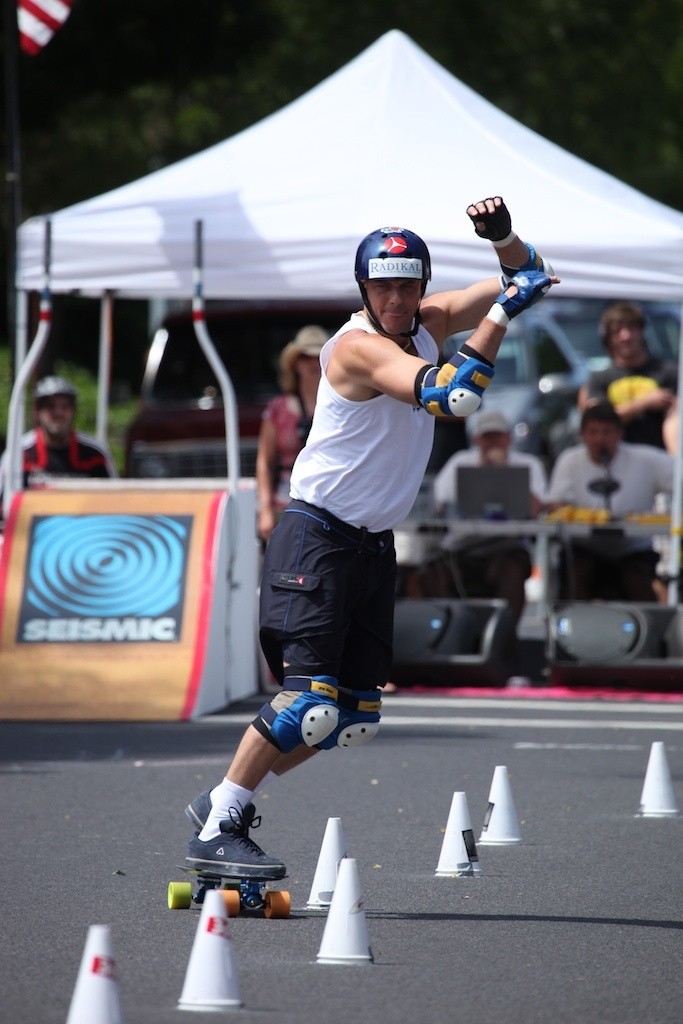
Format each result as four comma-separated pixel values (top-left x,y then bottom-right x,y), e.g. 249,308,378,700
34,375,78,398
354,227,431,282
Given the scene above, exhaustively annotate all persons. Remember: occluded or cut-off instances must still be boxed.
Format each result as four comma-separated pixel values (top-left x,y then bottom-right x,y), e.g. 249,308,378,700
575,301,683,457
432,411,546,615
547,398,676,605
255,327,330,542
0,376,112,483
181,197,562,875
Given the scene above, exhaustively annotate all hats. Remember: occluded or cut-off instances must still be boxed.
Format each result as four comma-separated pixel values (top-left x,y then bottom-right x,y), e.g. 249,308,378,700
280,325,330,391
474,410,508,439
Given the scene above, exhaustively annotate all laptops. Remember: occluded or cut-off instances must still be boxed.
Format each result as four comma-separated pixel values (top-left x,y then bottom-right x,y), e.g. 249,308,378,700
456,466,538,521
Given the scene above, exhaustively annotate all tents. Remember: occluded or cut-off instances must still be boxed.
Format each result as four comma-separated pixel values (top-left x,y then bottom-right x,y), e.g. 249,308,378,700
16,31,683,493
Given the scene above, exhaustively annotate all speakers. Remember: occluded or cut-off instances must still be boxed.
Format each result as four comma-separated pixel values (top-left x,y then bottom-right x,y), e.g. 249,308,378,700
545,599,683,694
388,599,515,689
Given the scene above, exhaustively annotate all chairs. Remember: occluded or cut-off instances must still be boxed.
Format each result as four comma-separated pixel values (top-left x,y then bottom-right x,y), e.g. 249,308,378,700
120,373,680,534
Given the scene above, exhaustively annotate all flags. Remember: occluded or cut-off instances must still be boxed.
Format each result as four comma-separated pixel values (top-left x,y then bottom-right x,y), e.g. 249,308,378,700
14,0,74,53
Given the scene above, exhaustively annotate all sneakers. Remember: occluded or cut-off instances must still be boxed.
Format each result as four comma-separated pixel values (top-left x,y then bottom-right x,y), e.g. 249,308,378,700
184,787,256,836
186,819,286,876
